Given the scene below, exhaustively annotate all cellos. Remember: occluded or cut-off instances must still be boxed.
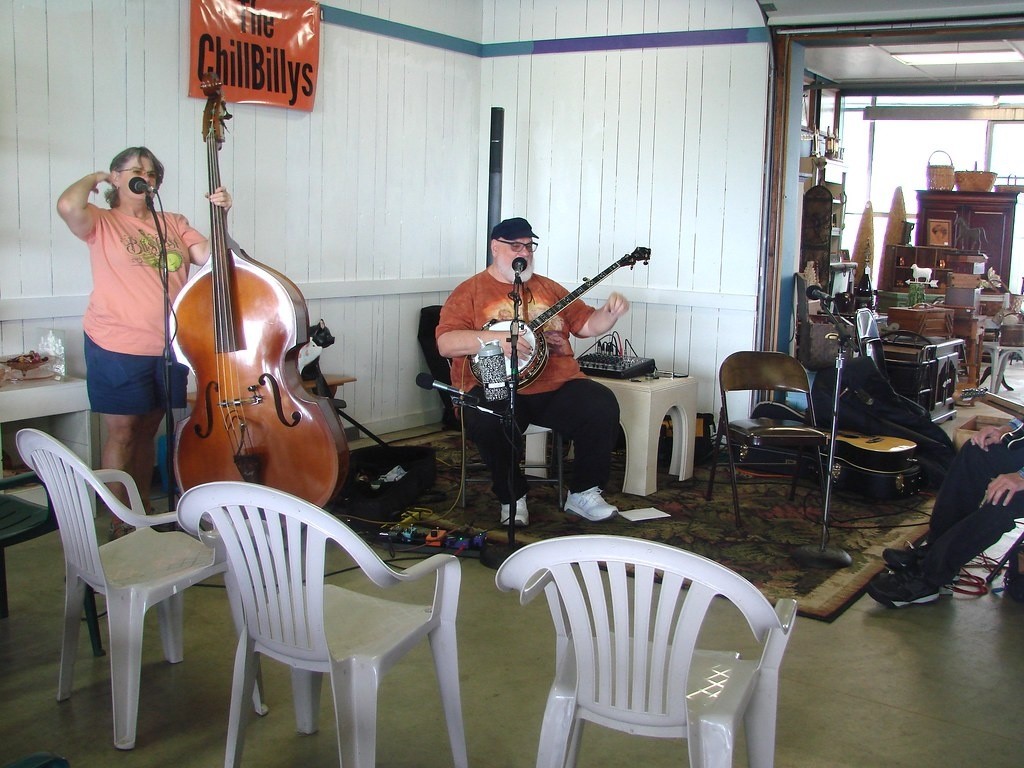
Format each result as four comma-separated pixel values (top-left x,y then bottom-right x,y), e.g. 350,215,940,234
167,73,348,532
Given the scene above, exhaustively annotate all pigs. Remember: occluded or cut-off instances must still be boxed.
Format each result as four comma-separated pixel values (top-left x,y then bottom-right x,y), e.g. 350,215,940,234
302,319,335,380
297,337,322,374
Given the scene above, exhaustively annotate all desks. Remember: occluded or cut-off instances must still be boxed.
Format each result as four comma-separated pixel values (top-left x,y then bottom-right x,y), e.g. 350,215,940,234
0,373,97,519
525,375,697,497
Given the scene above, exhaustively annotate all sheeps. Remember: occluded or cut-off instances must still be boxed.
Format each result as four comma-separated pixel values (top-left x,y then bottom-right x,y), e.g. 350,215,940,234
910,264,932,282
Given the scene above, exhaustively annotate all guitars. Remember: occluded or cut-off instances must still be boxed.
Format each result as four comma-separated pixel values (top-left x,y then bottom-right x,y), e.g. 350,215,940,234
467,247,652,394
814,426,919,474
954,385,1024,423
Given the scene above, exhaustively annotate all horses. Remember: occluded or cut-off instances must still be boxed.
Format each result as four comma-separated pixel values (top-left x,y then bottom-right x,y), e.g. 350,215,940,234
954,218,988,250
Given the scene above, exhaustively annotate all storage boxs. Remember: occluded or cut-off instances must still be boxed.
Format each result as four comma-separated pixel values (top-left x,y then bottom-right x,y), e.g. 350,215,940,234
946,255,987,275
796,273,856,371
954,415,1013,452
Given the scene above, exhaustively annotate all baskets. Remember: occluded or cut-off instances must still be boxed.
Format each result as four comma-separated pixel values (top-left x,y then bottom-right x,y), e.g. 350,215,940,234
926,151,955,192
994,174,1024,193
955,161,998,192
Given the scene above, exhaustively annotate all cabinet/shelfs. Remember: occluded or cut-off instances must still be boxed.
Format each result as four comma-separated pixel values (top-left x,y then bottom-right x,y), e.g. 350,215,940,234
915,190,1018,289
953,314,987,405
800,156,846,265
883,244,958,294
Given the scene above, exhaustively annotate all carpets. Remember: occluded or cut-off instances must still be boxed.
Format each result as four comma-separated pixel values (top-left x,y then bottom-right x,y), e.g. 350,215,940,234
347,428,939,623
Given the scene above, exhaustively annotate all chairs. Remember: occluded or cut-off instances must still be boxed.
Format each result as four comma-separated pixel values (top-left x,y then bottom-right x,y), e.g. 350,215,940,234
495,534,798,768
706,352,828,528
447,357,563,509
176,480,466,768
0,471,106,657
16,428,270,749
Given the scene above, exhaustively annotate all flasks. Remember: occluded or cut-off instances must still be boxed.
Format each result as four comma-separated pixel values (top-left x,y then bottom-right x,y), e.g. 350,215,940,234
477,340,509,401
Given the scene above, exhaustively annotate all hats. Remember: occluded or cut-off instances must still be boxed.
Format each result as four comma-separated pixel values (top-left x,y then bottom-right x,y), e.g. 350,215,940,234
491,218,539,241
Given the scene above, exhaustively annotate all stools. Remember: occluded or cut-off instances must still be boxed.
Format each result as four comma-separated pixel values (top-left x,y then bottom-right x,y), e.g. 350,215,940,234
983,342,1024,394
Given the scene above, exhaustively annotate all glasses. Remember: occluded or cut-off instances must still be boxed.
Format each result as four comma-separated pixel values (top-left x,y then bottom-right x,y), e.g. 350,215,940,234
496,239,538,252
115,167,161,180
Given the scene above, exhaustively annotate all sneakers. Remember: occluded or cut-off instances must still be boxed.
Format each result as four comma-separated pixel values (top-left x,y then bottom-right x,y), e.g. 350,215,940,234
563,485,618,522
866,539,961,609
500,492,530,526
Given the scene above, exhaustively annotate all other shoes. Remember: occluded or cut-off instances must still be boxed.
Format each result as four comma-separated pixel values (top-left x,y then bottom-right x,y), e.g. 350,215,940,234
147,506,171,532
108,521,132,542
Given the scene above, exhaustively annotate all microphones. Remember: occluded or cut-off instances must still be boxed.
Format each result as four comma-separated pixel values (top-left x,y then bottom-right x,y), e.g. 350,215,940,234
806,285,834,301
128,177,157,195
512,257,527,273
415,372,472,398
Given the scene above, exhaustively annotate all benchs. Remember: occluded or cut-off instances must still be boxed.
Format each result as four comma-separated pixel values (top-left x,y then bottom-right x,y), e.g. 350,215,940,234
184,373,357,412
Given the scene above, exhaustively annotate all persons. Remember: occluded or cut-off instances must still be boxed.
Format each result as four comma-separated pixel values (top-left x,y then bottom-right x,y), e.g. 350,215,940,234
868,412,1024,608
434,217,630,527
57,146,233,540
